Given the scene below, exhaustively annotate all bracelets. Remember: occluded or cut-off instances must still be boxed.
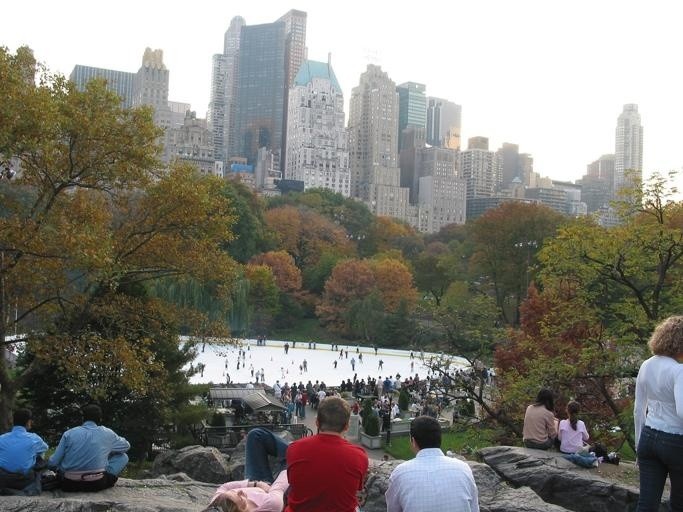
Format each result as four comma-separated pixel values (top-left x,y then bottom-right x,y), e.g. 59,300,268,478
252,478,258,486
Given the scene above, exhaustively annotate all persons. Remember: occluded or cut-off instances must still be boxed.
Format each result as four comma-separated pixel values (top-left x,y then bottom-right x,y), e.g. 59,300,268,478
203,426,292,512
521,388,558,451
554,400,592,455
632,314,683,512
385,413,480,511
188,333,498,462
45,402,132,493
281,395,369,511
0,406,49,496
